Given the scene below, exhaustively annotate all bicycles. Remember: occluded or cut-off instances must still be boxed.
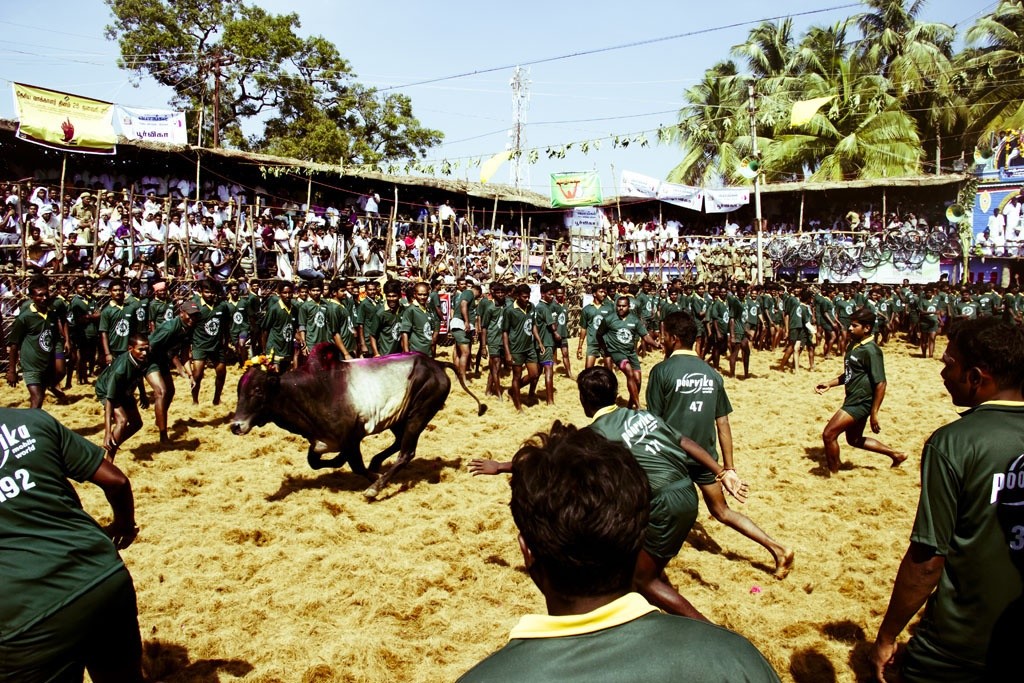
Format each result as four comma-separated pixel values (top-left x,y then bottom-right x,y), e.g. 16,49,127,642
861,226,962,271
750,231,866,276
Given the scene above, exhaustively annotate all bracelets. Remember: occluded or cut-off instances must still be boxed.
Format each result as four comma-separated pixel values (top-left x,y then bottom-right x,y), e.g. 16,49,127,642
714,470,728,481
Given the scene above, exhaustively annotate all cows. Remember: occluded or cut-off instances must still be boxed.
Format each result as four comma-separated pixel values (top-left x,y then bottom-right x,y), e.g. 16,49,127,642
230,342,488,499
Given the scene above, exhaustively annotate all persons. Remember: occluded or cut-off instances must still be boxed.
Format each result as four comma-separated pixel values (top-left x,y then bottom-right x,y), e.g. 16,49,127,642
94,332,154,464
456,418,783,683
646,311,795,581
814,309,909,473
0,407,143,683
0,169,1024,448
872,314,1023,683
466,366,751,623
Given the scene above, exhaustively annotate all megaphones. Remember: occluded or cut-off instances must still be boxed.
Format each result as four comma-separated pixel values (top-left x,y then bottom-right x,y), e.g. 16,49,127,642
740,155,762,178
946,204,965,224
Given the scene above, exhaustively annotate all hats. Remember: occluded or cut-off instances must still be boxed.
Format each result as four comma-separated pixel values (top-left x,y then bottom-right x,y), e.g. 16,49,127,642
180,302,202,316
